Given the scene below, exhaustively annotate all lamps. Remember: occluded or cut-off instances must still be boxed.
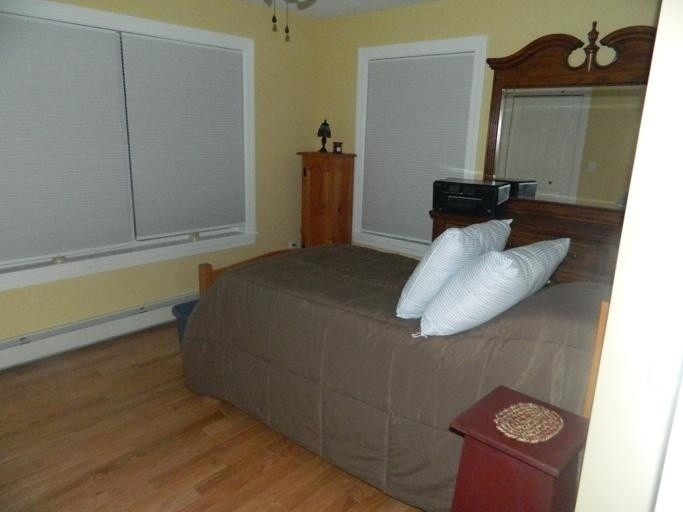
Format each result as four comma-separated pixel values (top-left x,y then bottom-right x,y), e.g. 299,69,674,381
316,120,330,152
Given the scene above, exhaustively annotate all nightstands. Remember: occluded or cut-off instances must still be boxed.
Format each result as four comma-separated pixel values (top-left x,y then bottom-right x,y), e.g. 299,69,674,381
447,388,589,512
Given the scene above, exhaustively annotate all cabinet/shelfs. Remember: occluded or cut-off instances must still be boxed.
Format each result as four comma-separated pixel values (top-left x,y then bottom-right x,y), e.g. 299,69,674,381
428,202,626,286
296,152,357,248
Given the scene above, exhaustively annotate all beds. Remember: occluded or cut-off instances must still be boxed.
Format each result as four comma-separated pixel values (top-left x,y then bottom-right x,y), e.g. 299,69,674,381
172,247,610,512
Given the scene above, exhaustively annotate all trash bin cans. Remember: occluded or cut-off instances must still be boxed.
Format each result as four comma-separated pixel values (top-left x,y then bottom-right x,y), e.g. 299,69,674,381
172,298,200,345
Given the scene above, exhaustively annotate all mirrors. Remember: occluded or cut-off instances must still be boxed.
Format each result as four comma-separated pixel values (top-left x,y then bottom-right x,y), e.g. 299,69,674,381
483,21,656,224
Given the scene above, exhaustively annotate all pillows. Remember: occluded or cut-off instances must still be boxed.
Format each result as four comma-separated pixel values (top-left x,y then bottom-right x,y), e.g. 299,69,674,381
395,217,513,319
411,238,573,334
